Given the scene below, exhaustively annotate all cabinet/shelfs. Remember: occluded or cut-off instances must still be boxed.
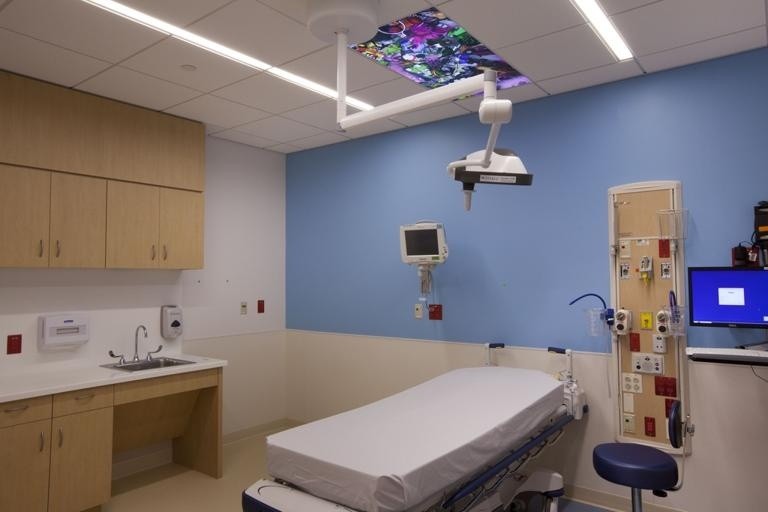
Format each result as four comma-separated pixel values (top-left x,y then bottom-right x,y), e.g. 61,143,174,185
2,386,112,511
2,71,205,192
106,178,206,268
1,163,107,270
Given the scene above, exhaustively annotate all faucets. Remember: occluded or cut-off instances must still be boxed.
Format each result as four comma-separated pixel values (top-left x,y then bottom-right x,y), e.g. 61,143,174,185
131,325,148,361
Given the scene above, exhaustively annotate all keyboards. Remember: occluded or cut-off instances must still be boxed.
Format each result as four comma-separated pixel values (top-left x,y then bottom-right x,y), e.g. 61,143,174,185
685,346,768,357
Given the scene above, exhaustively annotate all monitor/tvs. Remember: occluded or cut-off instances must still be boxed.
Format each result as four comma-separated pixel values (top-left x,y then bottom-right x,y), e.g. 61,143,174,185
400,223,449,264
688,266,768,330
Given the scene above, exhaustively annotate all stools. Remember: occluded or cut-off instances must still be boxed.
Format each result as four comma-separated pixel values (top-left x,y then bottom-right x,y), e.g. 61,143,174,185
591,441,679,510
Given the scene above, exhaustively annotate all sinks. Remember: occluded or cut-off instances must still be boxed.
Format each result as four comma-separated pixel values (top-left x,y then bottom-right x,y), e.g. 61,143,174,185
102,357,198,372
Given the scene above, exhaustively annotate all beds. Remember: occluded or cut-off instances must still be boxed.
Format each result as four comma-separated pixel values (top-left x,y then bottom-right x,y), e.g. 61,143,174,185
240,365,587,510
